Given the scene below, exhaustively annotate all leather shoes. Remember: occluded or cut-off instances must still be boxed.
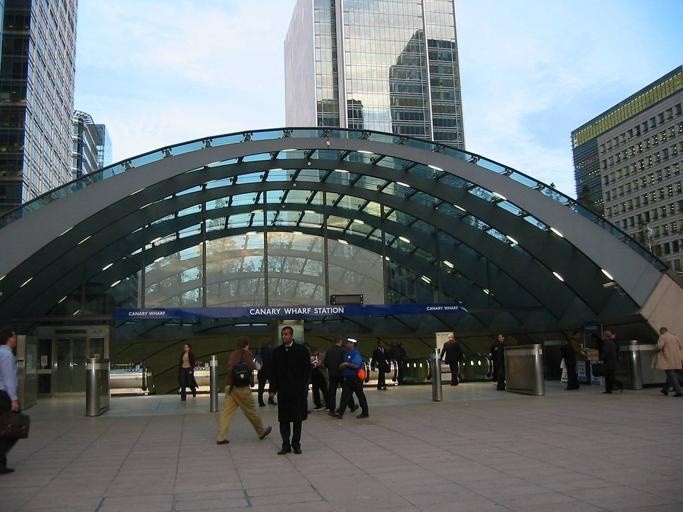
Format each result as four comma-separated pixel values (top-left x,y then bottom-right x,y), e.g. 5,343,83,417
661,388,683,397
259,427,272,439
294,449,301,454
278,448,291,455
217,440,229,444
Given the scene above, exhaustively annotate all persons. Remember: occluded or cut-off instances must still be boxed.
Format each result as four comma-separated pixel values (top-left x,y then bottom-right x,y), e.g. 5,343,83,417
490,333,504,383
179,343,199,402
592,328,624,394
257,336,370,419
649,327,683,398
269,326,310,456
440,334,463,386
0,327,21,474
373,338,390,391
216,336,273,445
563,330,592,390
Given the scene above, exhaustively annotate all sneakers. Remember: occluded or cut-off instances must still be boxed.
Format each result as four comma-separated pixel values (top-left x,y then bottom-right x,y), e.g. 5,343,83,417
259,404,266,407
313,404,368,419
268,400,276,405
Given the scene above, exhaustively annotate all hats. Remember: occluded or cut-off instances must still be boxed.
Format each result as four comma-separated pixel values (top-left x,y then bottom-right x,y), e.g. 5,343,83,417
344,338,357,344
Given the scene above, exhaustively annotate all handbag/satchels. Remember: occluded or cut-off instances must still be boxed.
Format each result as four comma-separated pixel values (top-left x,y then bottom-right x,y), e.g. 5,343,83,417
592,361,608,377
232,362,252,388
0,411,30,439
